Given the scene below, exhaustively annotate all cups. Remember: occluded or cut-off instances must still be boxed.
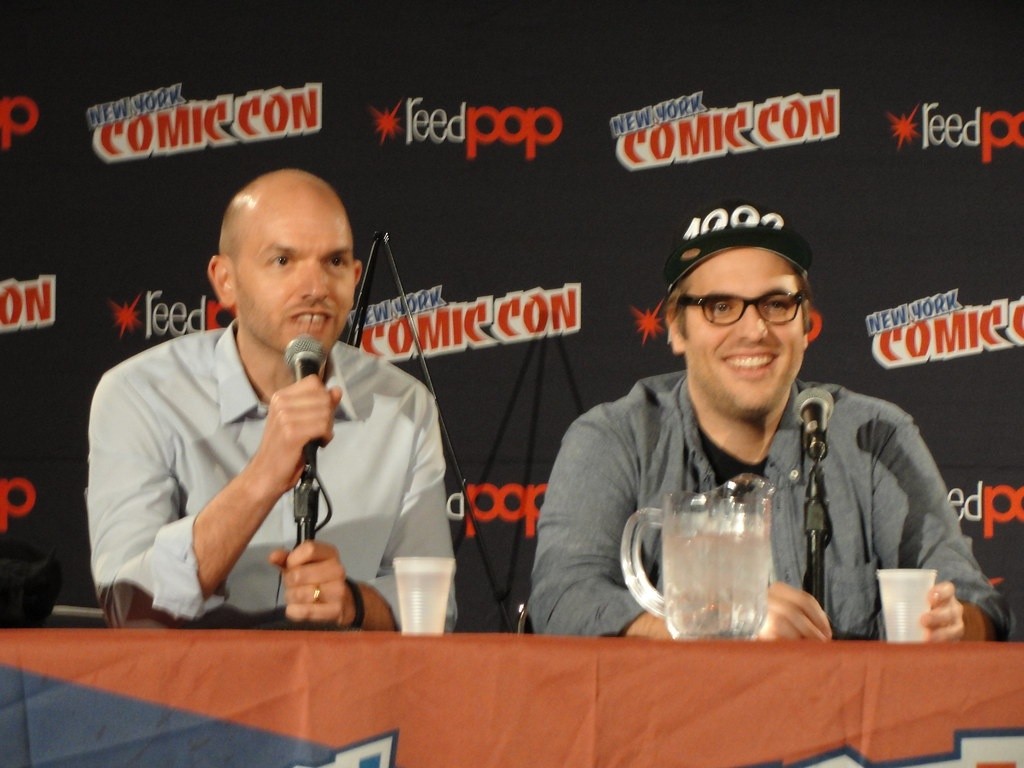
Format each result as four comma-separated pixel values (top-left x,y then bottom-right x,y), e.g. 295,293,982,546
874,567,938,645
391,556,455,638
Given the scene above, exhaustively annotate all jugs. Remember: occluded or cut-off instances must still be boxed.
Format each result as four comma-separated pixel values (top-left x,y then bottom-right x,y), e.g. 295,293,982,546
619,474,775,643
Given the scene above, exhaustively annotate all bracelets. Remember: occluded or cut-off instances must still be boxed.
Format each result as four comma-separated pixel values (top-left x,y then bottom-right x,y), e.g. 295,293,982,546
344,578,371,629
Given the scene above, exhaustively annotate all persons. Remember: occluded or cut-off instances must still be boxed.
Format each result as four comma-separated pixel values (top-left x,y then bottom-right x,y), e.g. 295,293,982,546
529,203,1023,643
83,173,461,634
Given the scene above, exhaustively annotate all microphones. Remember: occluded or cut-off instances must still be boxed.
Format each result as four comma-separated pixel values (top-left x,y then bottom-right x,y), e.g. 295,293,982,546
283,333,328,485
793,387,834,461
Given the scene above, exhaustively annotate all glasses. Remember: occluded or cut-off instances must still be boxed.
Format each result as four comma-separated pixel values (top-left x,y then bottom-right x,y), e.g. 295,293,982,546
677,292,805,326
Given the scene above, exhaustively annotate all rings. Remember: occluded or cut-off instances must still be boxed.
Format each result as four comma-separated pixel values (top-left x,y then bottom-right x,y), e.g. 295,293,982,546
313,584,323,604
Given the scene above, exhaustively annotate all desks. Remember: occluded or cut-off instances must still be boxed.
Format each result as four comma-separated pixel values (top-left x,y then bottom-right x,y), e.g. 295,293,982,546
0,624,1023,768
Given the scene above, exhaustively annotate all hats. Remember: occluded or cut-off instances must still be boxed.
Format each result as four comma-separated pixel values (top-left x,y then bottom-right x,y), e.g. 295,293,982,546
662,196,813,289
